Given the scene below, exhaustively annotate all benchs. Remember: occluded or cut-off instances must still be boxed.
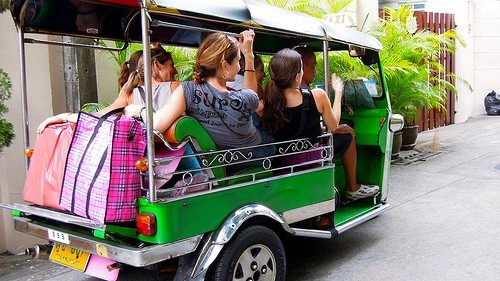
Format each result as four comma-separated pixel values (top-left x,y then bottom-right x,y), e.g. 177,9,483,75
80,101,272,192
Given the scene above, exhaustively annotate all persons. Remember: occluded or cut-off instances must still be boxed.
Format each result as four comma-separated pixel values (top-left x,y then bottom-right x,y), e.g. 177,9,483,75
37,28,380,201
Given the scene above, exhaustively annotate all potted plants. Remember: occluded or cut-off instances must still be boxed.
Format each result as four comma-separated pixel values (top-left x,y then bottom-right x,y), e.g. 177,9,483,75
310,0,474,161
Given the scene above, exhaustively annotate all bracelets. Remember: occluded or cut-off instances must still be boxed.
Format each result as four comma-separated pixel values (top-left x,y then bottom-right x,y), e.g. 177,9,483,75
242,69,256,72
66,112,72,122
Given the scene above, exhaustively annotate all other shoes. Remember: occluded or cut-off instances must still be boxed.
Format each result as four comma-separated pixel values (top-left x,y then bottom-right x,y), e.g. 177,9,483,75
346,183,380,201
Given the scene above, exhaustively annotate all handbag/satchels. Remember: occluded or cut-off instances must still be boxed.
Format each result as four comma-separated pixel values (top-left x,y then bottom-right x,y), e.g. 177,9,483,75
58,108,144,222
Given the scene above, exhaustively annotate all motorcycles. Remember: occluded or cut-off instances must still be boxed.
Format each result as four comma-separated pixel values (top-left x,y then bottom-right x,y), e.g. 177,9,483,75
0,0,405,281
